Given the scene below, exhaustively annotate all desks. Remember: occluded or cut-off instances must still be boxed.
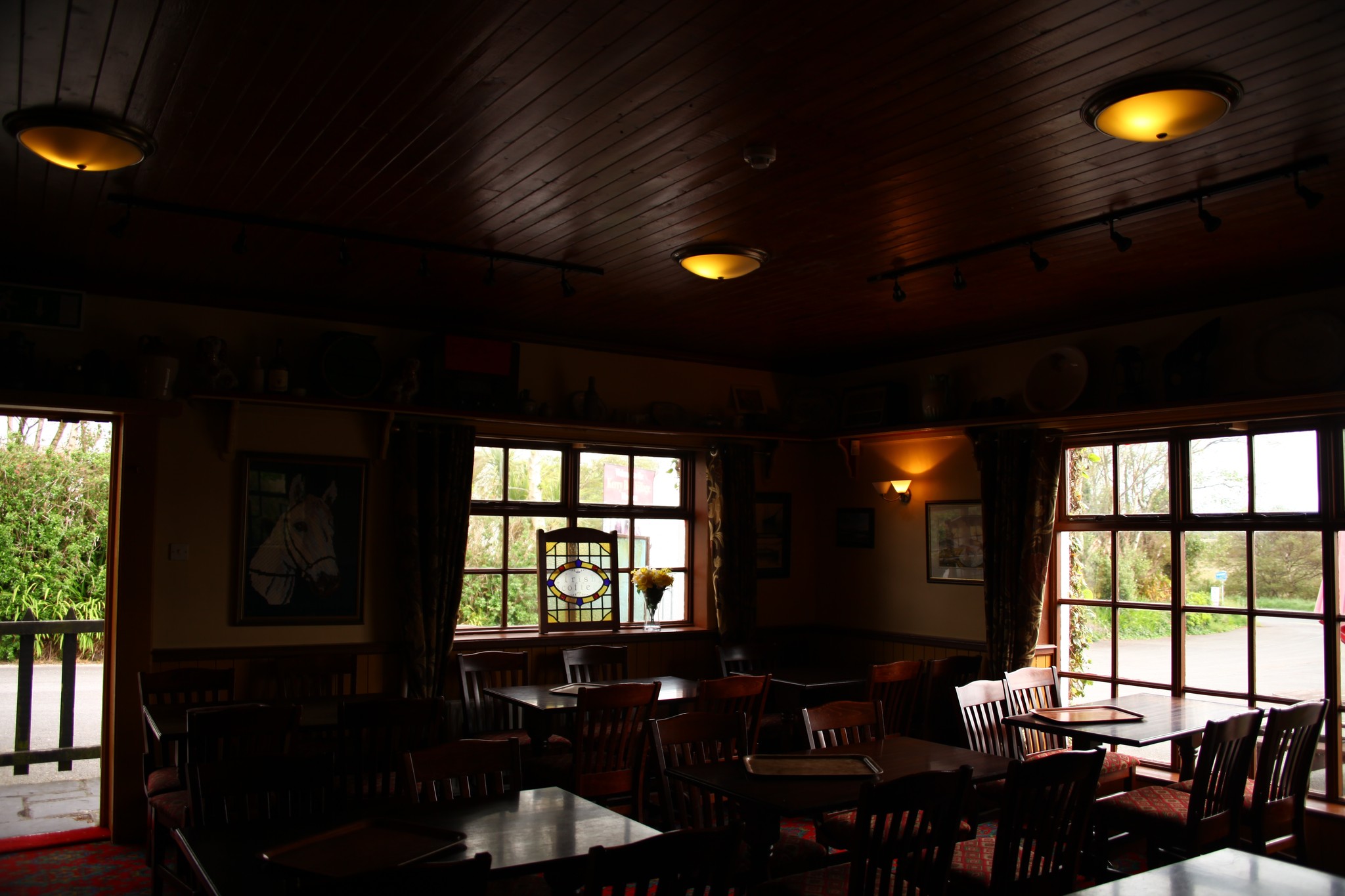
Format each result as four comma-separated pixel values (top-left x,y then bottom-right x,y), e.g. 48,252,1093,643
144,691,446,795
172,785,665,896
728,666,868,721
482,677,696,774
666,736,1022,896
1001,697,1270,758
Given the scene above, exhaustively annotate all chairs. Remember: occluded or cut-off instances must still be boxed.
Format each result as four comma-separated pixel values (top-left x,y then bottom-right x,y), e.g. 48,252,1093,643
136,642,1330,896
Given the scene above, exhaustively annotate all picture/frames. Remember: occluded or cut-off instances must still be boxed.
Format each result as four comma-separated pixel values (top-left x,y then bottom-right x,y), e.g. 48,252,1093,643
230,450,369,625
755,493,792,578
926,500,984,586
833,507,874,548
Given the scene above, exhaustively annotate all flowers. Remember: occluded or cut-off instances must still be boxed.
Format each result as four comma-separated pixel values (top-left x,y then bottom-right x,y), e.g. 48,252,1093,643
630,566,673,593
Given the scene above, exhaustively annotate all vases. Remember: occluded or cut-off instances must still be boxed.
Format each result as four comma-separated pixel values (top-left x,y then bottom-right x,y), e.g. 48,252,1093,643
644,588,662,630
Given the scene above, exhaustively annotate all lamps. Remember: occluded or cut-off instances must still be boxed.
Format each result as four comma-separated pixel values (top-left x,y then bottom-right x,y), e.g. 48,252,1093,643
108,191,603,297
2,104,154,172
873,480,911,503
865,157,1330,301
671,244,769,279
1080,70,1243,142
